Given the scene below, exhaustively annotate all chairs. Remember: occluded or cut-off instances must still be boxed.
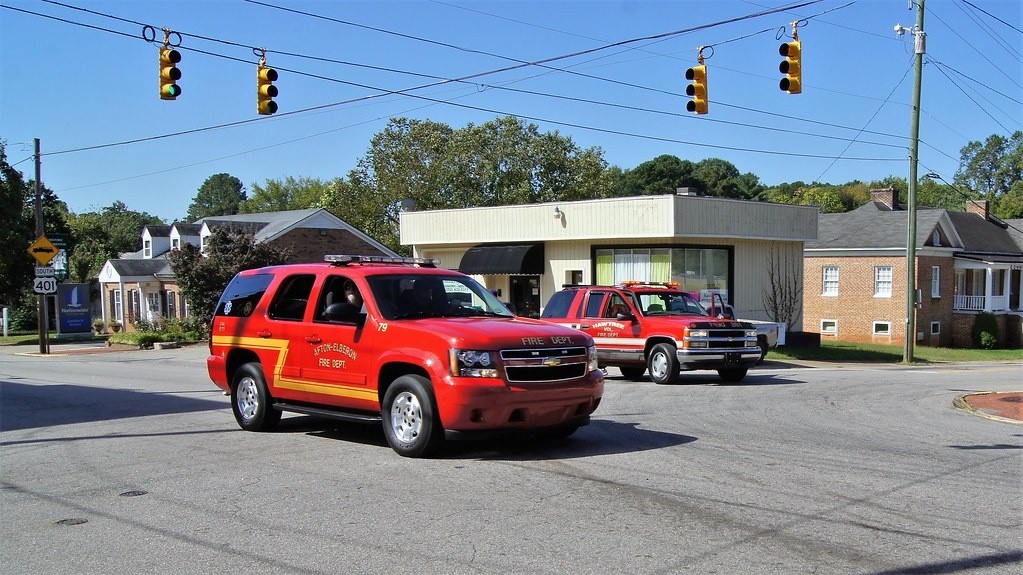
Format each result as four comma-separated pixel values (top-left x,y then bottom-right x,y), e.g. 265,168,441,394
609,304,627,318
648,304,665,313
326,290,348,308
397,289,431,314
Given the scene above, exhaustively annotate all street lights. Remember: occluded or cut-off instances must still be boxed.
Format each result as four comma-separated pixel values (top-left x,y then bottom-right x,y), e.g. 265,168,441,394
893,24,923,362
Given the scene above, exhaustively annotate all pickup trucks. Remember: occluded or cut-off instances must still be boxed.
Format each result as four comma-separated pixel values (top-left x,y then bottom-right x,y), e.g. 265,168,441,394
669,301,778,364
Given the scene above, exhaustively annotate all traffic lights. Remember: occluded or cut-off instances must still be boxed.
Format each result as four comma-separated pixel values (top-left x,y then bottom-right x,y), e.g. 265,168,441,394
161,47,182,101
780,43,801,94
686,65,707,115
257,67,278,116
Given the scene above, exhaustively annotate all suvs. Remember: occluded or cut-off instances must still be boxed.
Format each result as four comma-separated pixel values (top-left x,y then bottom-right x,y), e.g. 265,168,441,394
538,282,764,384
208,253,606,457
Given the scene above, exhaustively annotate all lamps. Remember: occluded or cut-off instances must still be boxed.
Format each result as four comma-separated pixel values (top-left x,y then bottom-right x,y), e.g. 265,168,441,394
916,288,922,310
554,206,562,219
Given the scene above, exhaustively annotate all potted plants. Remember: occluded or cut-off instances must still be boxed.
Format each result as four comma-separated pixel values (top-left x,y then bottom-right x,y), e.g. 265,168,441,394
93,318,122,336
